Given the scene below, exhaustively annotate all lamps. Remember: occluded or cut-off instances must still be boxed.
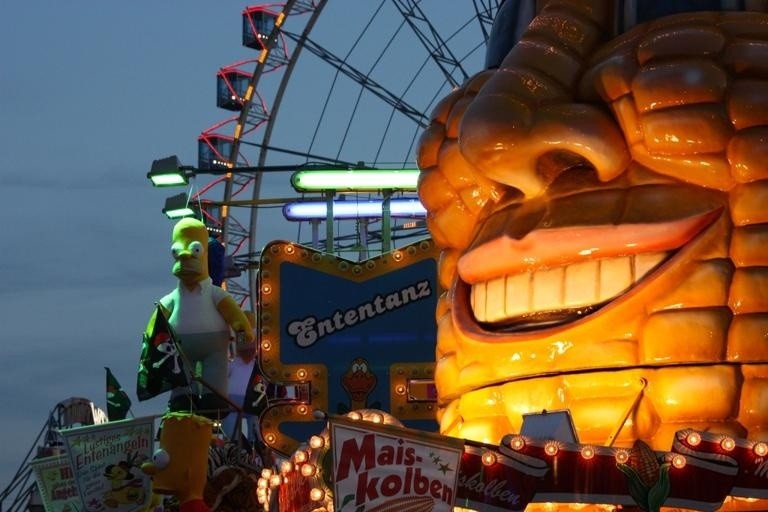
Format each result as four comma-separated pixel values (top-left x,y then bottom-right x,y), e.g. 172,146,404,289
145,155,200,222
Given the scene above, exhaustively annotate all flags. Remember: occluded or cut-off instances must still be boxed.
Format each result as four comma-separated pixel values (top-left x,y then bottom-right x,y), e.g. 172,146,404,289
135,308,192,402
103,368,132,422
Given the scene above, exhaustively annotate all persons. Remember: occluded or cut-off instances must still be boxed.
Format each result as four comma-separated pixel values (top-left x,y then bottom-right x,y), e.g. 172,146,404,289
418,0,767,453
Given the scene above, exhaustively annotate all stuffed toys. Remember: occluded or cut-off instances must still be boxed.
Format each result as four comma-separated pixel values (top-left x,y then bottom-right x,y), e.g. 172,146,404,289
141,412,219,512
141,218,255,415
